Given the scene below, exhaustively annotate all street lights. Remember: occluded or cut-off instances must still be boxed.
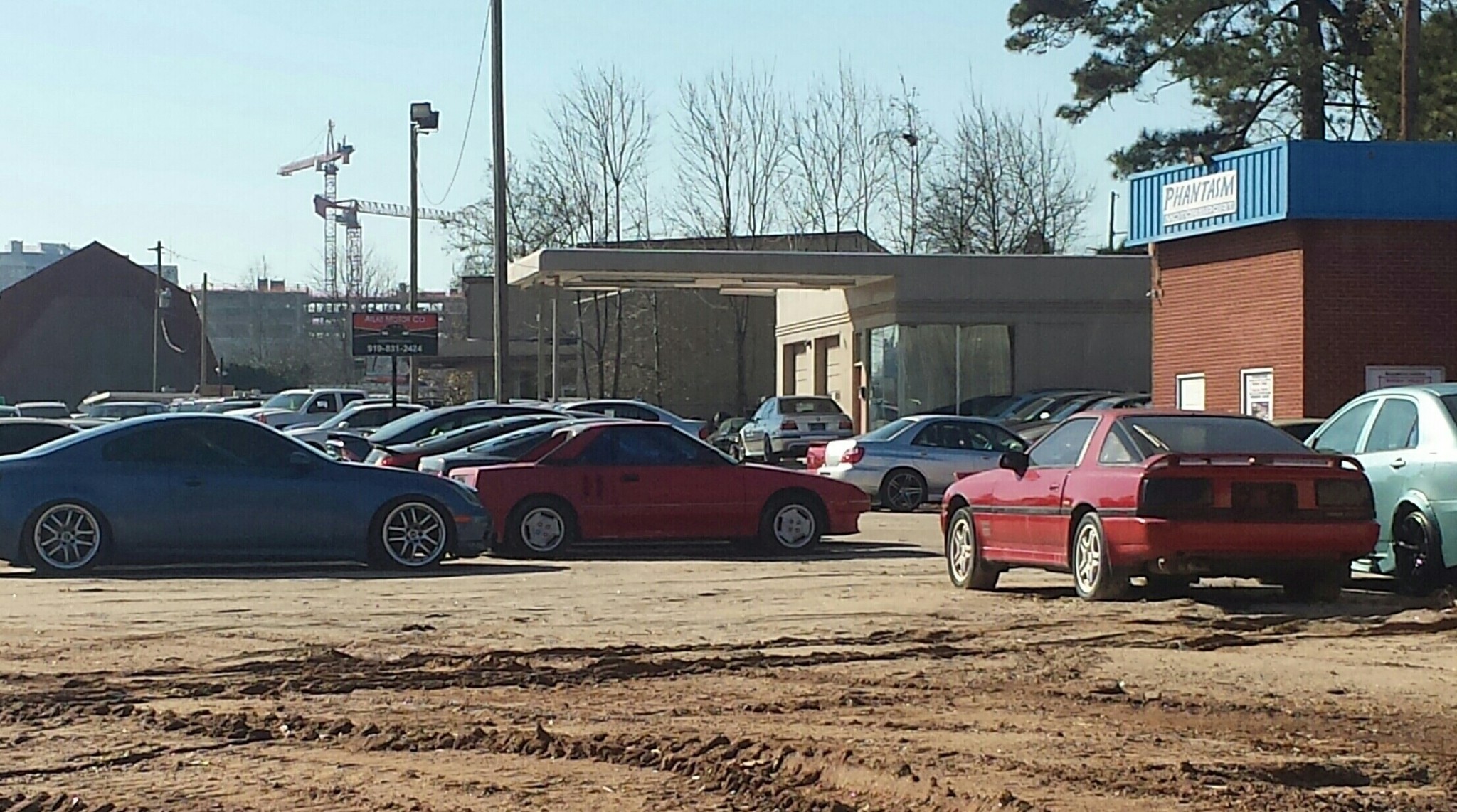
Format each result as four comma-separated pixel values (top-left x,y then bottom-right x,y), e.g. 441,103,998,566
409,101,441,404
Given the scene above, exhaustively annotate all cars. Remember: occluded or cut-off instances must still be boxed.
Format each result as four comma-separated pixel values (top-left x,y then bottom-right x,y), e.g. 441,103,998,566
806,379,1457,601
1,381,870,569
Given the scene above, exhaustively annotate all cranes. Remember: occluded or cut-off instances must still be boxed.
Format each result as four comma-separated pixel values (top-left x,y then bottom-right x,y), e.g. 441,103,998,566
312,192,462,295
279,118,353,293
